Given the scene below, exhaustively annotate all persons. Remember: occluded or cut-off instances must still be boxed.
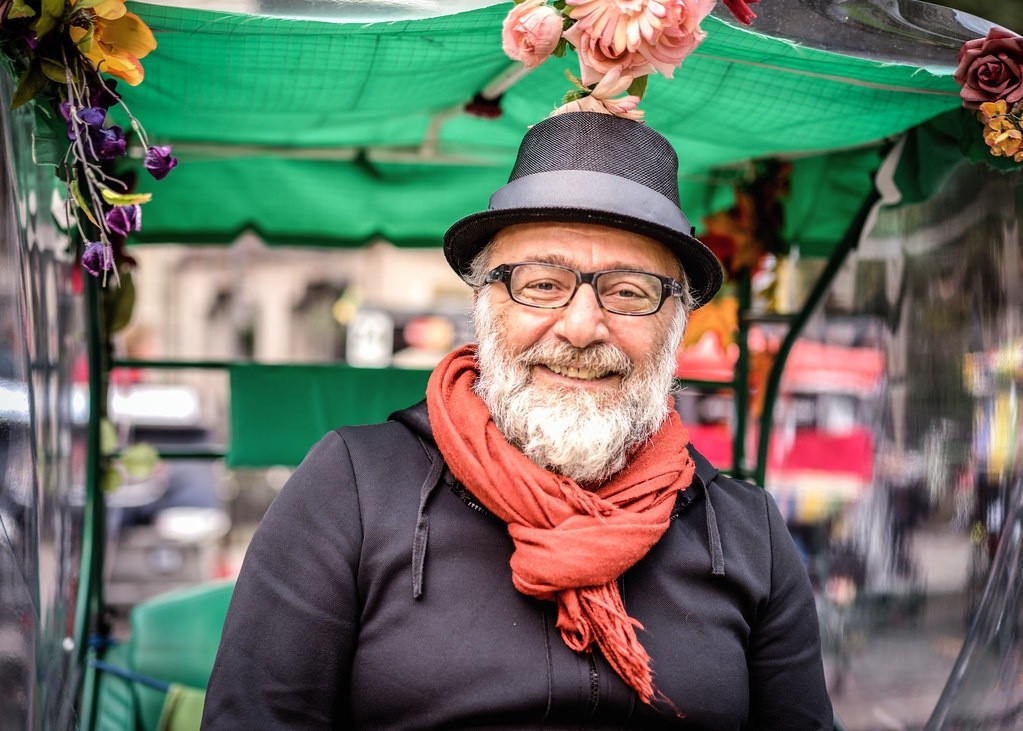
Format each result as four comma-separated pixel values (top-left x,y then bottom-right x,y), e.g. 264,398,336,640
199,112,839,731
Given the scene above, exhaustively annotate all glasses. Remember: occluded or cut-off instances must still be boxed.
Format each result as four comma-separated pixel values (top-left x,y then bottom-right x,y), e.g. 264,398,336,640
488,262,685,317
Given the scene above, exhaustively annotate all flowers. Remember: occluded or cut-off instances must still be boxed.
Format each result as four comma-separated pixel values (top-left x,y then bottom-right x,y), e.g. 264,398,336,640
502,0,719,128
0,0,176,291
953,28,1023,163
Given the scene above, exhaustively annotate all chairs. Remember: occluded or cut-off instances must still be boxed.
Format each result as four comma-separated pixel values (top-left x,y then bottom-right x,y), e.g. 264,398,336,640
94,584,242,731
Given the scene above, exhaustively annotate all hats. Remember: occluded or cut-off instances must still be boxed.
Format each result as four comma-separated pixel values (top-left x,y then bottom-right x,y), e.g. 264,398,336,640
444,112,723,310
